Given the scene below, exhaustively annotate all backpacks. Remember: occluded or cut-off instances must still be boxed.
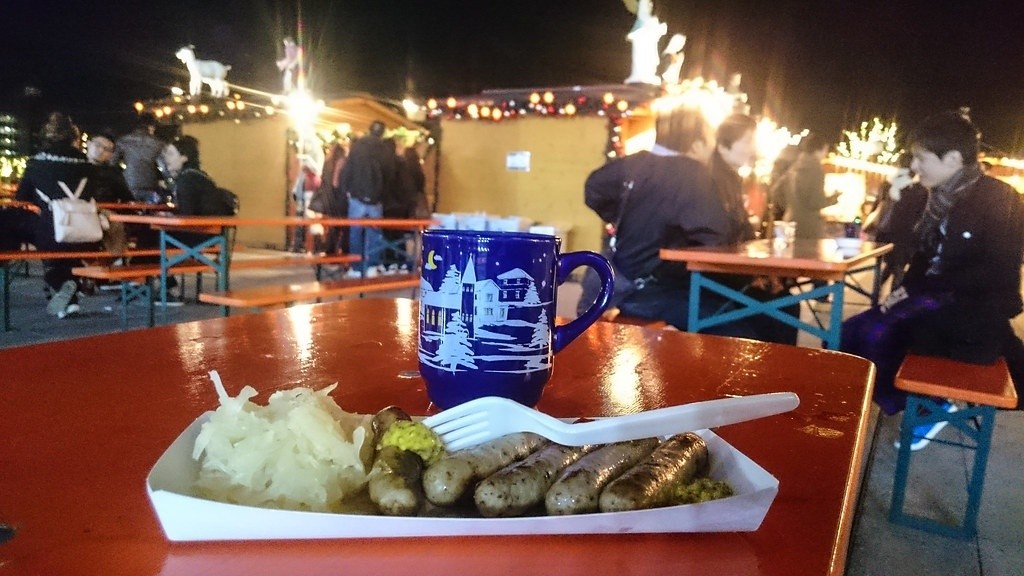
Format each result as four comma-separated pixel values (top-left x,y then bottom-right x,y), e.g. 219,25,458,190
36,182,105,244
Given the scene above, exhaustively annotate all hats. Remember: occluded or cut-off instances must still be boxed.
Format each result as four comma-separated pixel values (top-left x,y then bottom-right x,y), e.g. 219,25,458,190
42,113,79,142
171,134,199,161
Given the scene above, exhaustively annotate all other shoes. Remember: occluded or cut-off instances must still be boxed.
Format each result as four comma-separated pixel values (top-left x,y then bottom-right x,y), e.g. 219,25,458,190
342,263,409,279
47,280,80,317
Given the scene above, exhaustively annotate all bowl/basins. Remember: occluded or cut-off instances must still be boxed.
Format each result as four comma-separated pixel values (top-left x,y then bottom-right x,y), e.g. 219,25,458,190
836,237,862,249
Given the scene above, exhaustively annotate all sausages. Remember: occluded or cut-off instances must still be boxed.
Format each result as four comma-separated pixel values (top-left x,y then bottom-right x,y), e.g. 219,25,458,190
366,404,708,515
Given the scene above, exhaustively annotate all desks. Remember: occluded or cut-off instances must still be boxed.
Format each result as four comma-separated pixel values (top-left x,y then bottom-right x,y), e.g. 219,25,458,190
111,212,427,330
660,231,895,376
0,247,234,309
0,195,176,329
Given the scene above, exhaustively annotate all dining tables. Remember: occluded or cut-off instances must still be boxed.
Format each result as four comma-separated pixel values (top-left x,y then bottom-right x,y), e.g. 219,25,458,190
0,297,879,576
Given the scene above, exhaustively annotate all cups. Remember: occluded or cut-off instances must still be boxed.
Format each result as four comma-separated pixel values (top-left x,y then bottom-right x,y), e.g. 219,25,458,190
773,220,796,243
419,231,614,409
147,191,160,204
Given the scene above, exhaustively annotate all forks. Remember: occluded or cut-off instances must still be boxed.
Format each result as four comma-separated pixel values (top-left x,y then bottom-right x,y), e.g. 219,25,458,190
422,392,801,450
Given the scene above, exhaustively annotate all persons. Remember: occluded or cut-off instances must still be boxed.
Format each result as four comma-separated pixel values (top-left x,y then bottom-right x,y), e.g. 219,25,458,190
17,112,429,317
585,105,1024,449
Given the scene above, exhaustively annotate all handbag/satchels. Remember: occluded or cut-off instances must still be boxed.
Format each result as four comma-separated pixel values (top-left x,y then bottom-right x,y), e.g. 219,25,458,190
218,187,239,211
309,191,334,214
861,184,889,235
576,244,638,317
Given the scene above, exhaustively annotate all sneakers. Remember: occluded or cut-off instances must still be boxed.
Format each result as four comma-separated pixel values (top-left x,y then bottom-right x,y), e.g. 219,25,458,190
895,399,958,451
153,292,184,307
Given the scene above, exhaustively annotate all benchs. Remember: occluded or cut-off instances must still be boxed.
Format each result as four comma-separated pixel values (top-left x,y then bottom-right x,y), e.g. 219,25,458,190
72,253,364,326
200,271,422,312
886,350,1019,541
